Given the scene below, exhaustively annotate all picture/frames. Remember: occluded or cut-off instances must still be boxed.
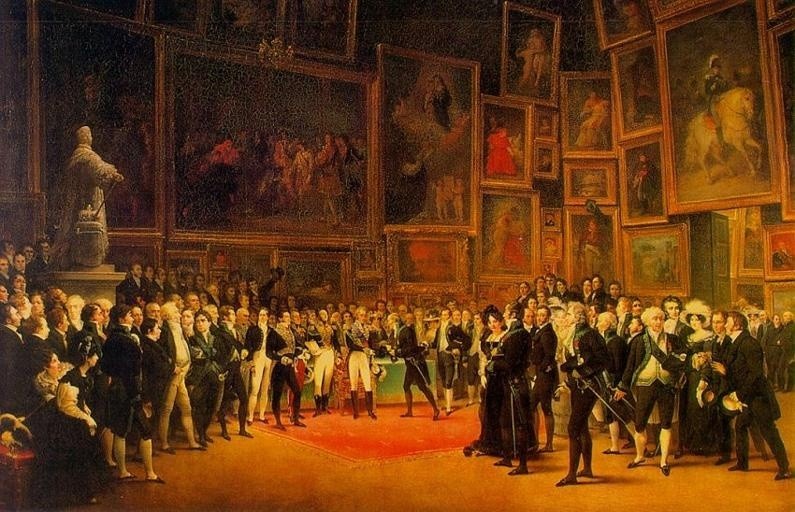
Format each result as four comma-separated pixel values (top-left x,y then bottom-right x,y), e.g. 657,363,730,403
0,1,795,326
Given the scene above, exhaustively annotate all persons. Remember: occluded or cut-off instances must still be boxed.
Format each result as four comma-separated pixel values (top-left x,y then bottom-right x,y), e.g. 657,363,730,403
1,0,794,504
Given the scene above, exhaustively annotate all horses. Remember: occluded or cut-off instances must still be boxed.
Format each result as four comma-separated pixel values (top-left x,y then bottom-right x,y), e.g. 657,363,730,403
685,86,763,185
